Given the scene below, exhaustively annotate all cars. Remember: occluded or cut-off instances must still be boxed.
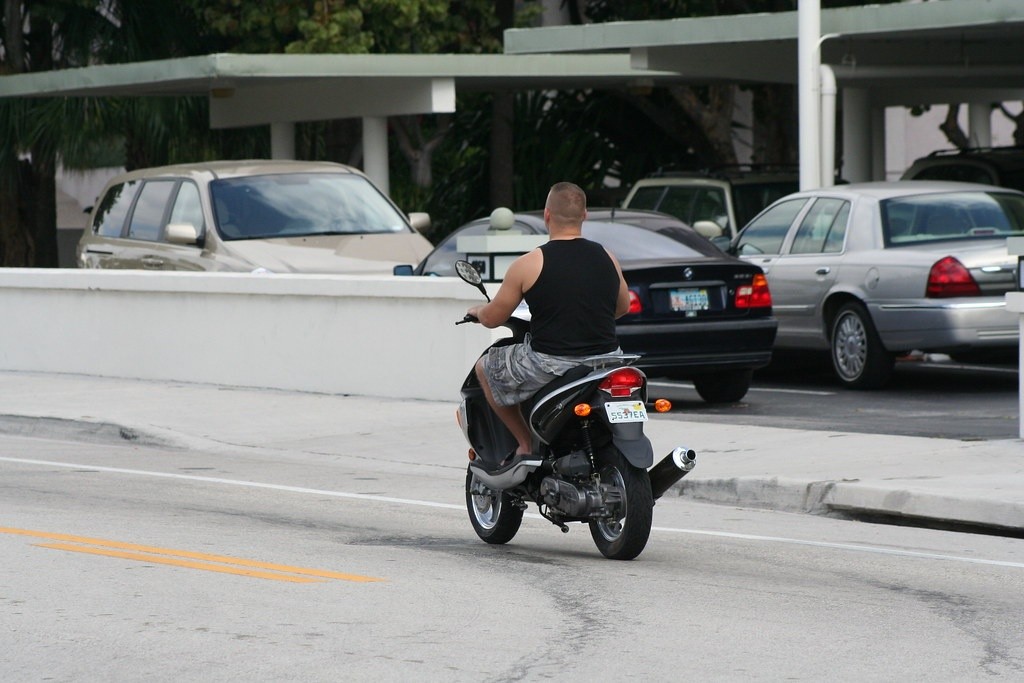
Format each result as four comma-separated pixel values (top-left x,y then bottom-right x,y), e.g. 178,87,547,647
75,159,452,280
392,206,778,401
710,181,1024,390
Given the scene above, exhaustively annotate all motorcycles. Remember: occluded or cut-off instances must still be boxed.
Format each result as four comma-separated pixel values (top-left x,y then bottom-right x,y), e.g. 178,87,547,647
455,259,697,560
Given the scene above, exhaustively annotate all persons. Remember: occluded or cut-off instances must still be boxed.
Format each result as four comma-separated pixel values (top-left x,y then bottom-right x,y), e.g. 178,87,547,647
466,181,631,458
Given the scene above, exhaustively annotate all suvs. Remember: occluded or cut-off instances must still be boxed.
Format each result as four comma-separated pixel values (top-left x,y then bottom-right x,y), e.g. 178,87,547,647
899,144,1024,184
620,166,849,247
